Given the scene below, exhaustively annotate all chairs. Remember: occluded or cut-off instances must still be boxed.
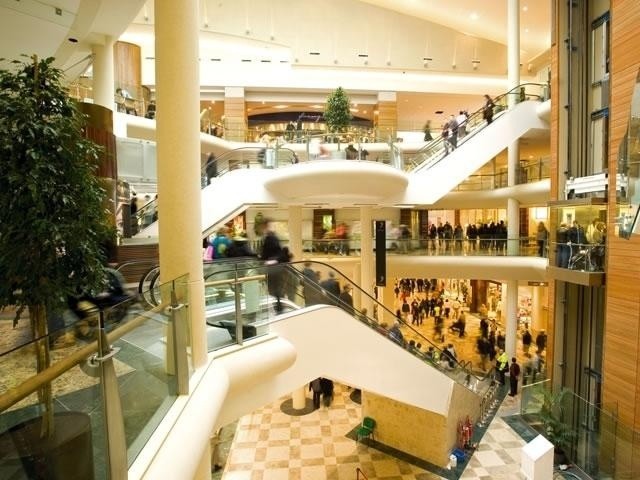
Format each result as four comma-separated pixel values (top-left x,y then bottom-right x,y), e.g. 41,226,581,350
355,417,375,447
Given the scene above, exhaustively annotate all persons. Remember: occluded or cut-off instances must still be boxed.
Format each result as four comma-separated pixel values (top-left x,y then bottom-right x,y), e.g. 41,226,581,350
385,277,466,369
422,94,494,156
428,220,605,272
131,192,160,230
116,87,224,138
201,211,387,336
475,320,548,397
206,152,218,184
345,141,369,161
307,377,335,410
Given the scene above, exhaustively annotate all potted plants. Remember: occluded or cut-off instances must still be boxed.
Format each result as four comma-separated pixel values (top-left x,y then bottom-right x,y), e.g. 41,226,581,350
2,48,129,480
535,385,583,465
322,85,353,159
